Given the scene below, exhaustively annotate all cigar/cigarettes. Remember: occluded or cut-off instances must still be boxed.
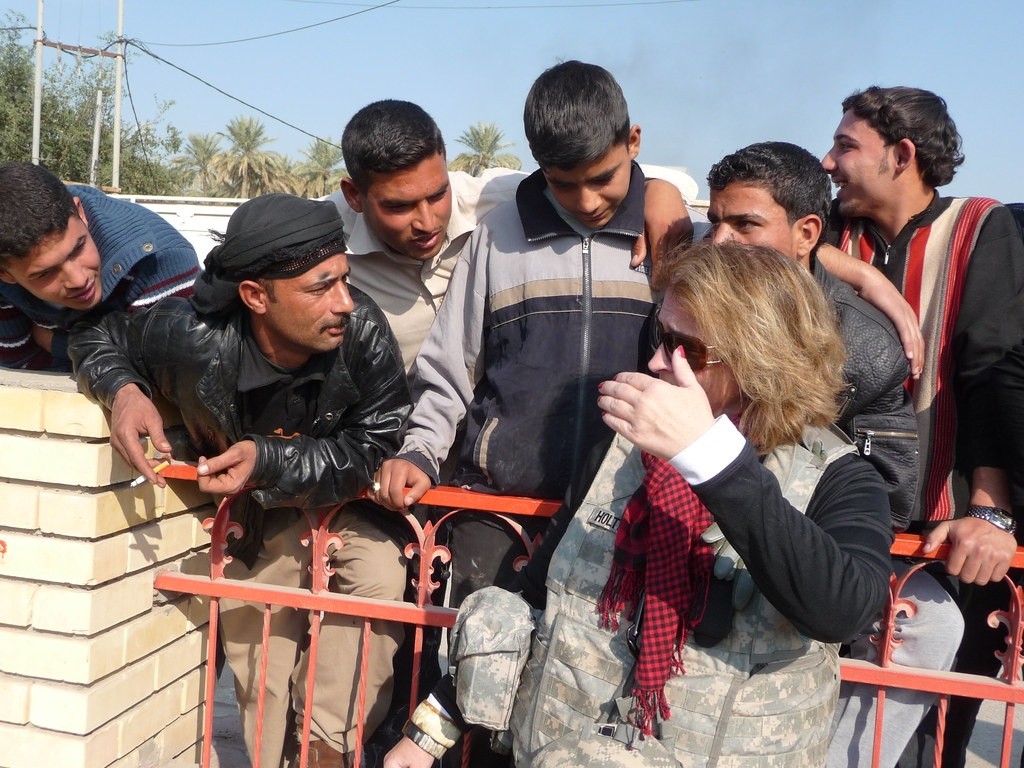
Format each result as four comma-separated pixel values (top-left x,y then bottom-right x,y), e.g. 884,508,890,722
131,460,170,487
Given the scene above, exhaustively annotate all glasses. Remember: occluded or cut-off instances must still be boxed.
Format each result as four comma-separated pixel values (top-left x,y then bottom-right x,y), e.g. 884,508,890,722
650,308,723,372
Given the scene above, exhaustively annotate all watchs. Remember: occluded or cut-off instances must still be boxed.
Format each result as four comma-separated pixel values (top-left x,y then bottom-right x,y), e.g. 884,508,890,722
964,504,1018,535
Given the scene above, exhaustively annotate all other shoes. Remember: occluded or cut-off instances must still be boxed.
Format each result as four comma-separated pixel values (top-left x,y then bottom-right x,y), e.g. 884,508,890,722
291,744,356,768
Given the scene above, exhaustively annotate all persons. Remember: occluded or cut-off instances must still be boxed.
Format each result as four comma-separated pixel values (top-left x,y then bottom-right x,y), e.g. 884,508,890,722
308,58,1024,767
67,191,414,768
0,160,206,372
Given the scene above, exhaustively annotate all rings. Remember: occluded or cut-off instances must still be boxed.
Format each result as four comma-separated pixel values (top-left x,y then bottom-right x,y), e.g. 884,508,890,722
371,482,381,494
